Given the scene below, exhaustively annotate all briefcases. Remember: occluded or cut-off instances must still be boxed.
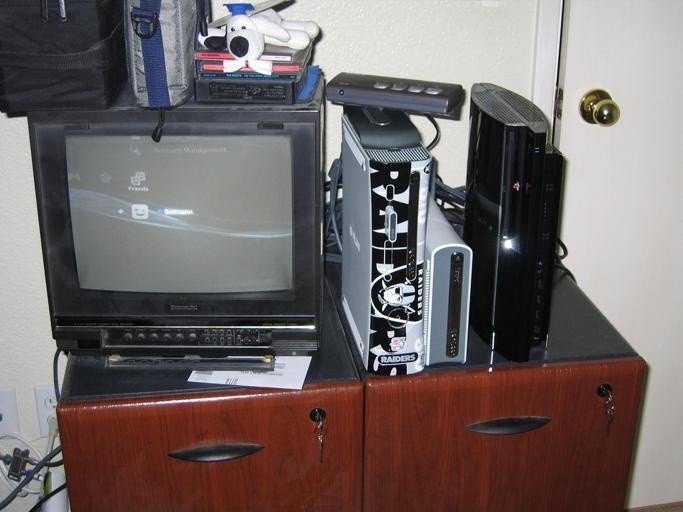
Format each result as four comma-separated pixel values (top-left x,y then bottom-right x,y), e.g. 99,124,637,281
0,0,128,113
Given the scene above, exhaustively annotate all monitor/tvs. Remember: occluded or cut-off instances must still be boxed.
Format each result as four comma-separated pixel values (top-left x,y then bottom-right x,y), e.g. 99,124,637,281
27,71,326,370
462,84,563,363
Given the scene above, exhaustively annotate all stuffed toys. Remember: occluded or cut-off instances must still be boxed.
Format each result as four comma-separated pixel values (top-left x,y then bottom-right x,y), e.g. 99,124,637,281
197,8,320,76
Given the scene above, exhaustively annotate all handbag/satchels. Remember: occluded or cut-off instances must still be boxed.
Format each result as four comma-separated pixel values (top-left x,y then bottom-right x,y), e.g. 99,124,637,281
123,0,211,143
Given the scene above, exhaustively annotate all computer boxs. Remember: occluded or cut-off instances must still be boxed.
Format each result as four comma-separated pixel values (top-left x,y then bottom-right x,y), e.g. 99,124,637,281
339,115,432,379
